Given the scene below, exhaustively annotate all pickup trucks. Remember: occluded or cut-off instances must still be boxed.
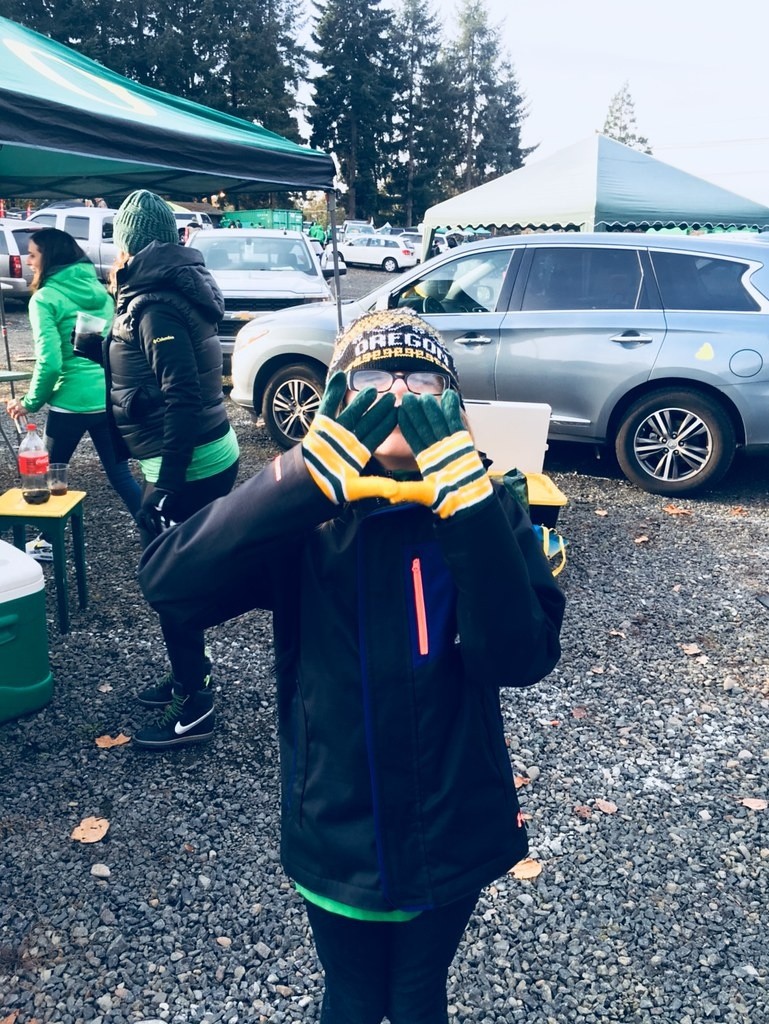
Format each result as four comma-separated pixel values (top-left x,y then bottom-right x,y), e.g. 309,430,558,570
25,208,119,284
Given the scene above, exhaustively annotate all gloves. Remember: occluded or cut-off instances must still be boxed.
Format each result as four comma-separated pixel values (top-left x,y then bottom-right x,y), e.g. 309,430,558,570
136,491,188,539
69,324,104,360
301,372,493,518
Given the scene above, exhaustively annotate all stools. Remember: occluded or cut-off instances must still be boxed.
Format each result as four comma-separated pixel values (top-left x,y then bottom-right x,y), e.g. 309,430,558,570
0,486,87,635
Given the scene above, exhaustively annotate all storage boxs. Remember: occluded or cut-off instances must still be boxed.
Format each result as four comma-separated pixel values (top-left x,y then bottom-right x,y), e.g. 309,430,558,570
0,536,56,723
485,472,570,534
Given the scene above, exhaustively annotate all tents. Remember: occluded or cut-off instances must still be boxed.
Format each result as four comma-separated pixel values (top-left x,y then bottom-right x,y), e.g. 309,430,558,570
415,134,769,265
0,11,347,483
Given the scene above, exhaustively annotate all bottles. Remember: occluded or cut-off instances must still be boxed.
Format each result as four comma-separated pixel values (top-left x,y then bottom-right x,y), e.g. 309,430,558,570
17,423,51,505
12,409,29,435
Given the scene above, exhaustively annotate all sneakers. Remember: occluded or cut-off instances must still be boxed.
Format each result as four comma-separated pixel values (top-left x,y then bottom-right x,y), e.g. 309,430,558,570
132,658,214,749
25,532,54,561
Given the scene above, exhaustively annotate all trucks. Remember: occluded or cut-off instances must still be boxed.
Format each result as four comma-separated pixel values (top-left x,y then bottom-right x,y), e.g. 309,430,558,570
171,212,215,232
219,209,305,234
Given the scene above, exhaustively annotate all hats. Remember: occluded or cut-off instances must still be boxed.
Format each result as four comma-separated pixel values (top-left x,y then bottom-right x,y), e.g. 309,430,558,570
112,188,178,256
327,307,467,409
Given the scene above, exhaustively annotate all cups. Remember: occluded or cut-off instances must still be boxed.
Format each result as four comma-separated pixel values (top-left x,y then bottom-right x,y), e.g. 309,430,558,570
47,462,69,495
72,310,108,355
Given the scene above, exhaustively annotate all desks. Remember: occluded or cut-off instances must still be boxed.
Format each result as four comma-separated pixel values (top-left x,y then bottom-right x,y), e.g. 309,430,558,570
1,369,34,478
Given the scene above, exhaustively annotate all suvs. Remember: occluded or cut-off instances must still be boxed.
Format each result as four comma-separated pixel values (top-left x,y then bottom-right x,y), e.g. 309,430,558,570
310,219,488,273
180,226,349,374
227,231,767,497
0,219,50,297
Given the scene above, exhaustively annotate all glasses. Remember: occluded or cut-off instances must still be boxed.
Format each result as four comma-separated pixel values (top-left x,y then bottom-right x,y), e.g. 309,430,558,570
346,368,452,396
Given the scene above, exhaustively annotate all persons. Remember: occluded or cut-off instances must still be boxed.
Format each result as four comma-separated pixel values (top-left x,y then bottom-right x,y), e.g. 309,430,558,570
2,229,163,542
72,188,236,746
133,305,571,1023
183,216,268,244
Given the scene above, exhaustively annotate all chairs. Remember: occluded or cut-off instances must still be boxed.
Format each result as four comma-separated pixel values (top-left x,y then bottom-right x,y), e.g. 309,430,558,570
203,249,233,270
274,253,301,270
